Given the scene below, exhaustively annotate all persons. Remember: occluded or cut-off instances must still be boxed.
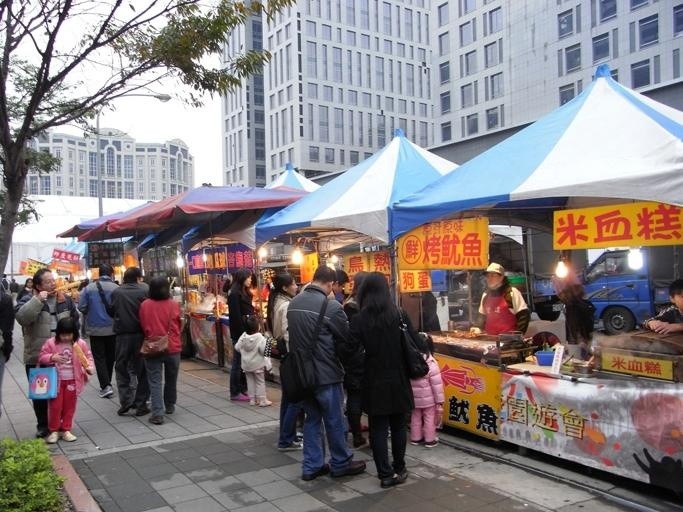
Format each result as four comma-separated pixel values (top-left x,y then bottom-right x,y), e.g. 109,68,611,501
77,264,120,398
326,270,347,297
345,272,429,487
234,315,272,407
15,277,34,336
420,291,440,331
227,268,254,401
267,271,304,451
110,267,148,416
14,270,80,438
342,272,368,451
408,331,445,448
139,278,181,425
286,265,367,481
470,262,530,335
39,316,94,444
642,277,683,335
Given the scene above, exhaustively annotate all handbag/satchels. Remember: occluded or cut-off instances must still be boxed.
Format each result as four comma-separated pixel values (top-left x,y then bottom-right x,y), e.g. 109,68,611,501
396,306,430,380
136,301,175,361
28,360,58,400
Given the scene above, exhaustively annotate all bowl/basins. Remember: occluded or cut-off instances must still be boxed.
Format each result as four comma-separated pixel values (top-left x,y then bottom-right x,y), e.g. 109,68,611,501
573,362,594,374
534,351,554,366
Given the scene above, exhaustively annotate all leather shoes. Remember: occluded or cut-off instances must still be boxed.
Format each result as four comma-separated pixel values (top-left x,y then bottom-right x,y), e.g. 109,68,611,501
329,460,367,477
380,472,408,488
301,462,330,481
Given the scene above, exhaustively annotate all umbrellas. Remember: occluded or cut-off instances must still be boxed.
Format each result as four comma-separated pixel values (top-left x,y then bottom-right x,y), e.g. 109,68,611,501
78,185,313,245
56,211,127,237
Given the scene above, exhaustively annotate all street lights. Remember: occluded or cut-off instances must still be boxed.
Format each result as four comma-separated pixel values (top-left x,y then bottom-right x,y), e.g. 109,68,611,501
96,93,171,219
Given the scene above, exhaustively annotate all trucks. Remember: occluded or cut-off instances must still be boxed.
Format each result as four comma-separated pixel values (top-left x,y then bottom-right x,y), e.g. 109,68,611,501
430,226,589,325
579,245,682,335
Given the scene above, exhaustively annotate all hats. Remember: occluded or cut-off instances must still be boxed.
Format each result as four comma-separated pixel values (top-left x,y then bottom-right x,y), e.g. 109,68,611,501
480,261,504,277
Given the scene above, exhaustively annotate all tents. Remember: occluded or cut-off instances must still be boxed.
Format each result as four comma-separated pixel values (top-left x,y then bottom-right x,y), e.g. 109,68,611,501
254,129,466,250
182,163,322,250
389,64,683,256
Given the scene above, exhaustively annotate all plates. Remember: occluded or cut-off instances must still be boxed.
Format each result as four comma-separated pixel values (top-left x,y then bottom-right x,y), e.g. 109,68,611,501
569,372,596,378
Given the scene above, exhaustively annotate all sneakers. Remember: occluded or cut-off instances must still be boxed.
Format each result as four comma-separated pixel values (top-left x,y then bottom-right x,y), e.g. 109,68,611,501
147,413,164,425
136,407,152,416
96,384,115,400
258,399,272,407
352,438,370,451
45,430,60,445
277,441,304,450
249,399,256,407
423,439,440,448
410,436,425,445
117,400,135,415
61,430,78,443
230,393,251,401
35,427,48,438
166,403,175,414
